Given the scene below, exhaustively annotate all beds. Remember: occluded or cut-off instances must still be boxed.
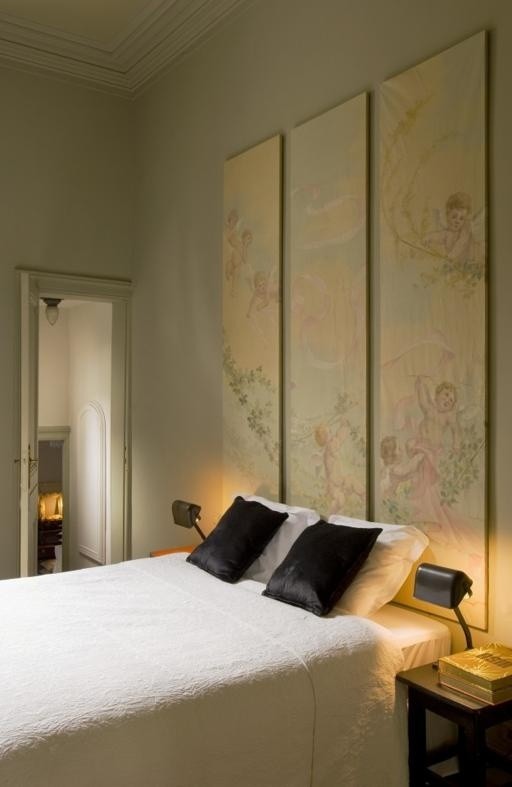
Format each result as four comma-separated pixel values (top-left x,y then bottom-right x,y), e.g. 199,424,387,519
3,489,484,785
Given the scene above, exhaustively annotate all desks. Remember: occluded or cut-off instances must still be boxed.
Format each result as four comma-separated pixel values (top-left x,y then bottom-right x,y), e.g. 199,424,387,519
396,651,511,781
148,541,199,557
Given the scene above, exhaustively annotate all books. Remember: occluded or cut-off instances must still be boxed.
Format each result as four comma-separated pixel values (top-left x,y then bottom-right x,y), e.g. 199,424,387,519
439,643,512,692
438,671,512,706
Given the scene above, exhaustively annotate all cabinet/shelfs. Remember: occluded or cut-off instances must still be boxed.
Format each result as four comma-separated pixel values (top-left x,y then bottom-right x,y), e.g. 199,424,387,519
37,518,63,561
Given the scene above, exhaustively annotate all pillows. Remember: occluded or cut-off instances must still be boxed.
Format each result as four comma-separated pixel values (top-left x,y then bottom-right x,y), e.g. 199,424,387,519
260,517,384,616
324,513,431,618
184,492,290,583
240,494,321,583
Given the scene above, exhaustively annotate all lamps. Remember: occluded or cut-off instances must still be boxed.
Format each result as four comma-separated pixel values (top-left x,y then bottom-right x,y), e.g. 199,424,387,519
41,296,65,327
169,496,208,543
412,560,478,649
